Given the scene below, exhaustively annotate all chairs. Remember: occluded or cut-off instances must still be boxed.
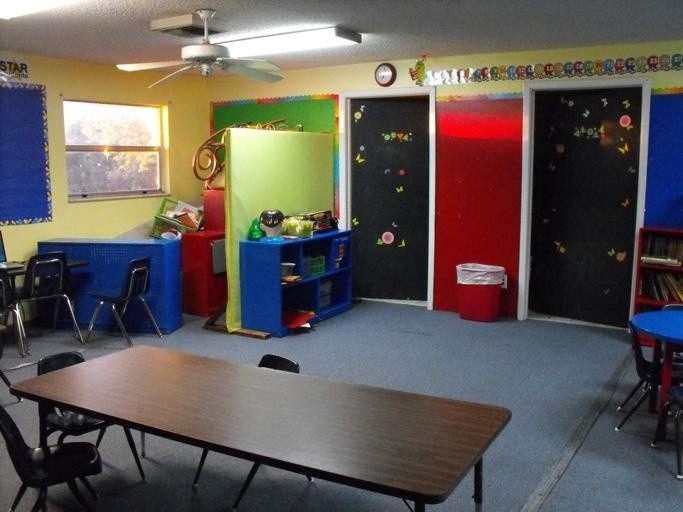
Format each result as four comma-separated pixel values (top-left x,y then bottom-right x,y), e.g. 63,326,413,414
615,322,682,431
191,353,313,510
26,352,149,485
662,304,683,312
649,385,683,479
1,268,26,364
85,255,162,344
2,404,101,512
11,252,84,355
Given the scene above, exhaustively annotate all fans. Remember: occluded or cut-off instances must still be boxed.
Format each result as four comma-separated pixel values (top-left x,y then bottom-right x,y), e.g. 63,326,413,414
116,40,286,88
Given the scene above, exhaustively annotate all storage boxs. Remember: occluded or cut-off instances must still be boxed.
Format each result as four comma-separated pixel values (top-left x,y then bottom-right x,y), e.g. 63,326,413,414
150,197,203,240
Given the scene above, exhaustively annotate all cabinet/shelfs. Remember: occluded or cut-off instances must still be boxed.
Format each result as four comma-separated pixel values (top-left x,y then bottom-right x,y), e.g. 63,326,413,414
634,225,683,345
240,230,354,337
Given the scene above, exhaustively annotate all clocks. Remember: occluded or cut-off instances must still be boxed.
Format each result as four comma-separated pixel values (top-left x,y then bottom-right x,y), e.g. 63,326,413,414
375,63,397,87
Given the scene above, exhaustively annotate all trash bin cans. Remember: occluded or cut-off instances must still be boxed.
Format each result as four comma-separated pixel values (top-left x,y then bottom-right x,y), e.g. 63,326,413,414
455,262,507,324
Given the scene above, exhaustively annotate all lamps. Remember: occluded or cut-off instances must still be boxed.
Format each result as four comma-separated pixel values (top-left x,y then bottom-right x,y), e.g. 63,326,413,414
202,64,214,78
188,26,362,58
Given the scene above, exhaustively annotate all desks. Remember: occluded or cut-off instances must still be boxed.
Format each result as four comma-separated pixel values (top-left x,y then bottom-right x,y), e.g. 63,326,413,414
11,346,511,512
38,237,182,334
631,310,683,443
0,259,90,340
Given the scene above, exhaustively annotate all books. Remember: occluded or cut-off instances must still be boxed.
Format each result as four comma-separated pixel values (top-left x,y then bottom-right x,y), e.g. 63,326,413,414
640,269,683,303
641,232,683,267
